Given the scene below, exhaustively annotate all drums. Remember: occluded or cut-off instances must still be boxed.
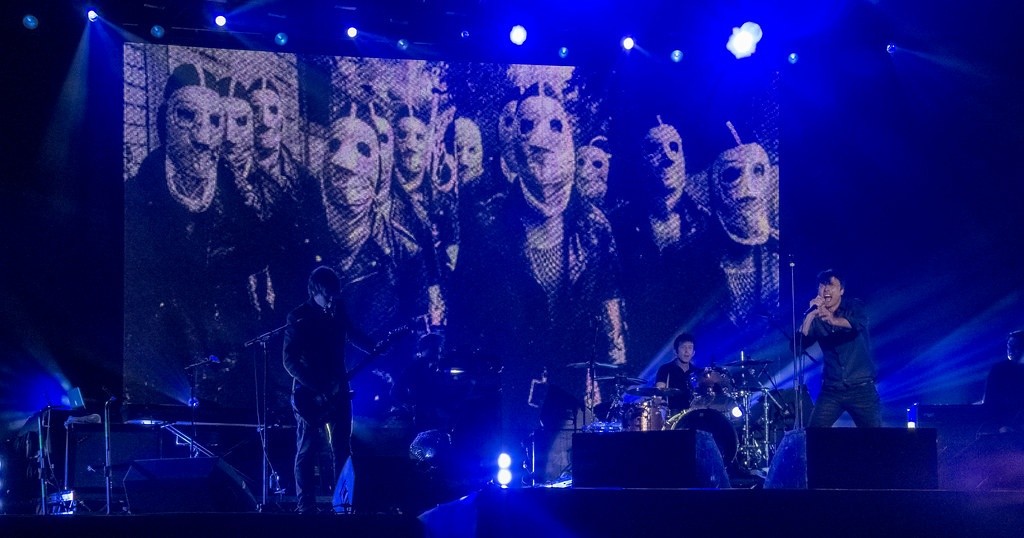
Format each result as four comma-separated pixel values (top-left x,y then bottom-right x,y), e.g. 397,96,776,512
628,395,740,471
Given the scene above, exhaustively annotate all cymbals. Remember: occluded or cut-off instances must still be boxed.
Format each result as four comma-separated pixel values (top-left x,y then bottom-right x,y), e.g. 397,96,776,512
723,357,775,368
566,362,648,386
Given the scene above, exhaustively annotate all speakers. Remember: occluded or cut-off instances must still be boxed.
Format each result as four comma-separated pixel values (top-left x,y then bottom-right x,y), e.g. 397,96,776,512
763,426,938,489
122,459,223,513
332,456,448,515
571,433,731,488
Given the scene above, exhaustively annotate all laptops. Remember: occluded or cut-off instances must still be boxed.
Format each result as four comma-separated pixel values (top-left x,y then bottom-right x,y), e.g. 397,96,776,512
66,387,86,410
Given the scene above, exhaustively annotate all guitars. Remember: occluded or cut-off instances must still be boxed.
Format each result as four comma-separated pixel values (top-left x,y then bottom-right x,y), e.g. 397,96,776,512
290,324,410,430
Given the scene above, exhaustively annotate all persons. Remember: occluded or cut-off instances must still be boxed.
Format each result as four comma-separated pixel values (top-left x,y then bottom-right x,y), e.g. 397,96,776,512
126,61,778,408
983,329,1023,406
790,267,883,429
654,334,716,416
283,266,355,515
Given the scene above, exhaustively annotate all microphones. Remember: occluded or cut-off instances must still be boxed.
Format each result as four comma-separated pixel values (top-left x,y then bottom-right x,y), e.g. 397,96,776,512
804,305,818,316
